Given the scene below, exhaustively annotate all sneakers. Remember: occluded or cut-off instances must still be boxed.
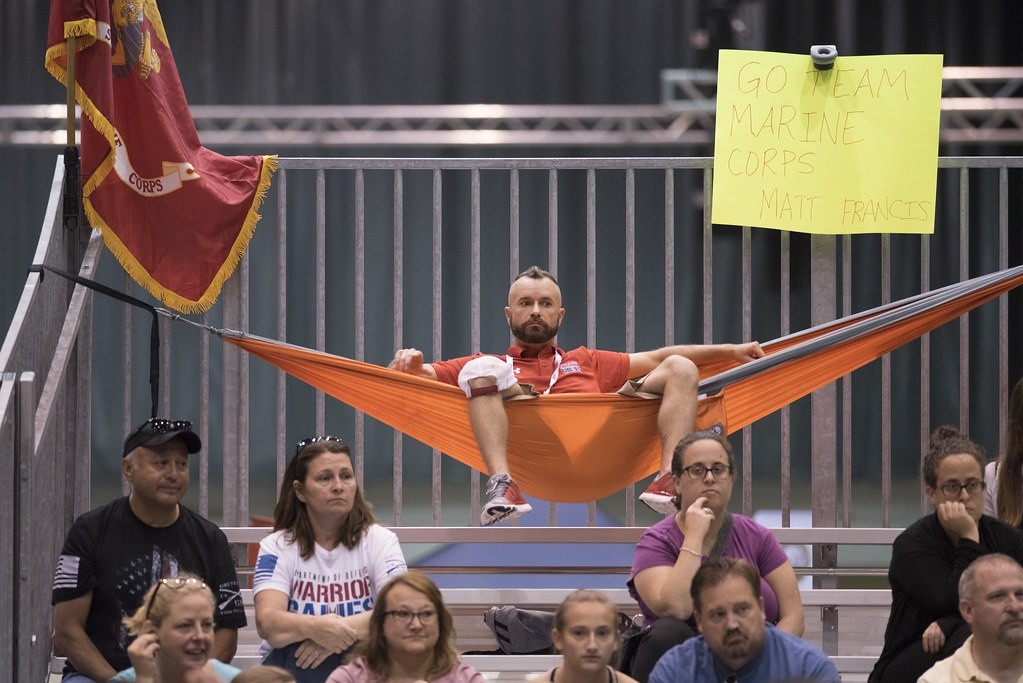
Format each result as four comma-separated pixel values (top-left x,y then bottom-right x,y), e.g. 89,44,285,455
480,475,532,529
639,477,679,515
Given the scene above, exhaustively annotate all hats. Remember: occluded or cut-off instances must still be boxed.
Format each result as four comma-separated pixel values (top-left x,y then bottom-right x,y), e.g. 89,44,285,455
122,423,202,453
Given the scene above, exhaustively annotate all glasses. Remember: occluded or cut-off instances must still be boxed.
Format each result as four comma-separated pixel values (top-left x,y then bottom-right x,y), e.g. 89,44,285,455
681,463,733,481
131,419,193,434
296,436,344,454
382,609,440,626
937,480,986,497
146,573,208,620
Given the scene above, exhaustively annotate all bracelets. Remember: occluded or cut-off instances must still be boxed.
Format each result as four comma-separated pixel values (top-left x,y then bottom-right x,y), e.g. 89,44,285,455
679,546,703,559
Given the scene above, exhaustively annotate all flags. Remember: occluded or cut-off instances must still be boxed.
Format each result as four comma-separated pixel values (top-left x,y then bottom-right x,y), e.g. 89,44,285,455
45,0,279,315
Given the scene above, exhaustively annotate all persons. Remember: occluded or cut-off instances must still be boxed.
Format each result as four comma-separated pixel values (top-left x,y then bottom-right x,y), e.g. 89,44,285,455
323,571,489,683
867,425,1023,683
917,555,1023,683
387,265,765,528
647,556,842,683
50,417,247,683
626,429,806,683
977,378,1023,529
252,434,409,683
520,589,644,683
107,571,243,683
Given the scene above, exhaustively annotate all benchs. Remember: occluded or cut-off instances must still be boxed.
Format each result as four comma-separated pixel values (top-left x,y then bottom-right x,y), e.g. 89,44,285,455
47,527,905,683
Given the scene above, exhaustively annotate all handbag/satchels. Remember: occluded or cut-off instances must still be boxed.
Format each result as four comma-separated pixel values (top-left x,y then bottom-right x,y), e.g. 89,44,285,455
483,605,559,653
